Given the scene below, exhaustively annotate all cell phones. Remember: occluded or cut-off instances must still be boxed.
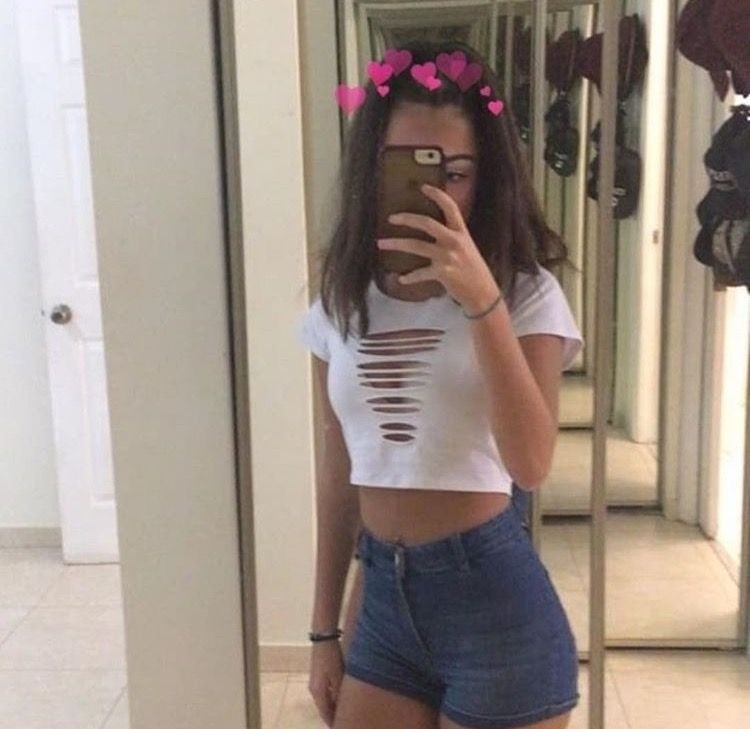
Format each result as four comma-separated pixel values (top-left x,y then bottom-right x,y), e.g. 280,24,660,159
377,145,446,274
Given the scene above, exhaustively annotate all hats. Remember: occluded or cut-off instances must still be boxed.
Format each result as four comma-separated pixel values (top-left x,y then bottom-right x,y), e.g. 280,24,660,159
677,0,750,286
513,12,639,221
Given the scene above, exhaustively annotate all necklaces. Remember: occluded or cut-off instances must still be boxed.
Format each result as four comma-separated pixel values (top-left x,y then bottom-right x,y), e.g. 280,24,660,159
380,260,431,276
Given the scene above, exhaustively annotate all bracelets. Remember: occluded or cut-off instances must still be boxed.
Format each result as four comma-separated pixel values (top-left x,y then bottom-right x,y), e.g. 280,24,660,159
309,629,344,641
463,292,503,320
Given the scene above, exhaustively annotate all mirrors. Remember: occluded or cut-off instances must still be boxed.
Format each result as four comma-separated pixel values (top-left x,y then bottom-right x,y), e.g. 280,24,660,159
216,0,620,728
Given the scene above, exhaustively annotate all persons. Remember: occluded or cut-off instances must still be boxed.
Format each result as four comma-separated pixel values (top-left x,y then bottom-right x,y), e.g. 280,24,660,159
309,42,586,729
